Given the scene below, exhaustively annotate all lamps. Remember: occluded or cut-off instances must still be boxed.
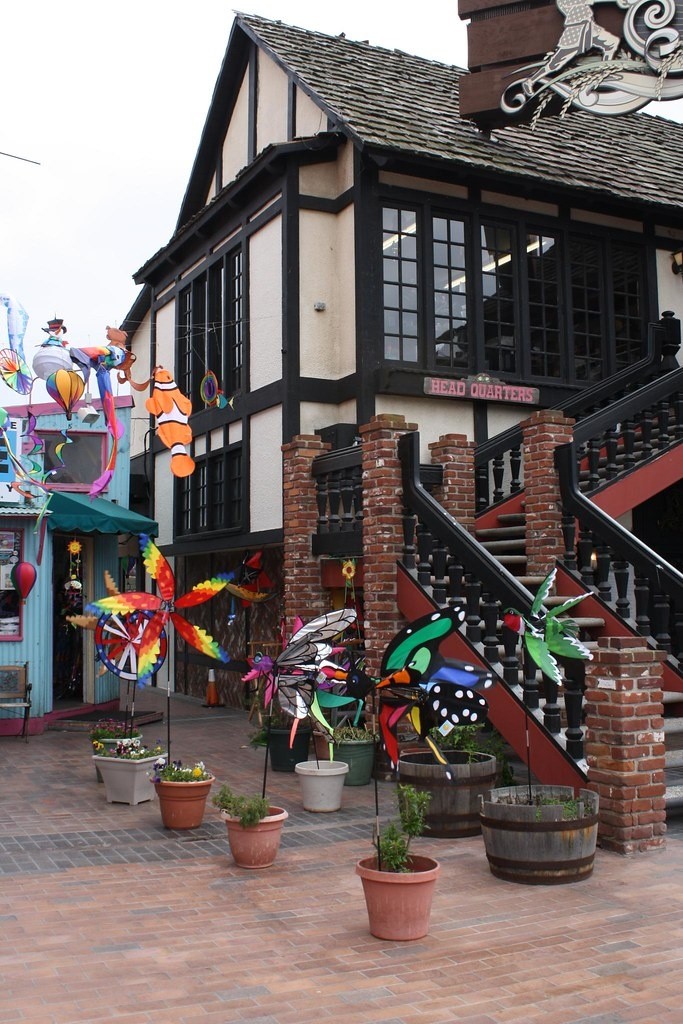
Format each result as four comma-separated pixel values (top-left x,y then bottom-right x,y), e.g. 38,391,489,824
671,247,683,281
77,403,100,423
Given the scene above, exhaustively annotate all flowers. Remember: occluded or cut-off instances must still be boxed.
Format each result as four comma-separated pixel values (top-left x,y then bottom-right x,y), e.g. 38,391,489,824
90,738,173,760
145,757,214,784
88,718,144,742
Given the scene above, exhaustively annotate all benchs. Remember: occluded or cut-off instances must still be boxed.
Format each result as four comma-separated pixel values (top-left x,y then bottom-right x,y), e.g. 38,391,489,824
0,660,32,744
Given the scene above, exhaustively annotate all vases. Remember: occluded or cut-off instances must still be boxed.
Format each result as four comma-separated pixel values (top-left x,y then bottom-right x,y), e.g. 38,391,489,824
95,734,144,784
146,775,216,830
92,753,168,806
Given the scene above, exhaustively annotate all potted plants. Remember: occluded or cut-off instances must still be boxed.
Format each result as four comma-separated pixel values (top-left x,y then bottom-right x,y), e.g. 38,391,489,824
246,710,312,773
333,720,381,786
211,782,289,869
398,739,497,839
476,785,599,886
354,784,440,942
